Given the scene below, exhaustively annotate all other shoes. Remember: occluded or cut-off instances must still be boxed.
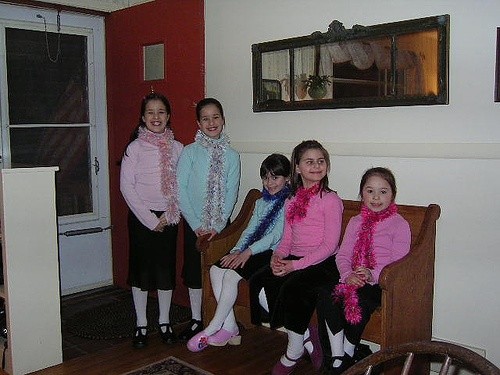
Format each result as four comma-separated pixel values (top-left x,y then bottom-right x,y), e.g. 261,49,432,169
272,346,308,375
178,319,204,342
327,352,351,375
133,326,148,348
343,345,359,369
207,329,241,346
303,325,323,369
158,323,177,345
186,330,207,353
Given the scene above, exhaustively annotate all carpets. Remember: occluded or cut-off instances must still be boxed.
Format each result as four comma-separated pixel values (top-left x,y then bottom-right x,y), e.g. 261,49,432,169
65,295,191,340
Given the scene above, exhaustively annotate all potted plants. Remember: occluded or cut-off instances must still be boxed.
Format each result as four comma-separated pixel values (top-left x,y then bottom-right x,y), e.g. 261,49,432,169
302,73,332,100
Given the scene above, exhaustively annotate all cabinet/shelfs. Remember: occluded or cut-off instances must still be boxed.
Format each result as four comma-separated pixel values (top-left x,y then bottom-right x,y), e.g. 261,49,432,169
0,166,64,375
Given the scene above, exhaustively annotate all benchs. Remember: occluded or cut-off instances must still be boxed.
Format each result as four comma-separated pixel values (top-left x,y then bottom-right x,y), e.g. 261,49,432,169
195,188,441,375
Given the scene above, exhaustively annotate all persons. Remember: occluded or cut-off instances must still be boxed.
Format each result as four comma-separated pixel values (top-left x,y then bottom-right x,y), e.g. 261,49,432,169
176,98,241,343
186,153,294,352
119,92,184,350
322,167,411,375
249,140,344,375
